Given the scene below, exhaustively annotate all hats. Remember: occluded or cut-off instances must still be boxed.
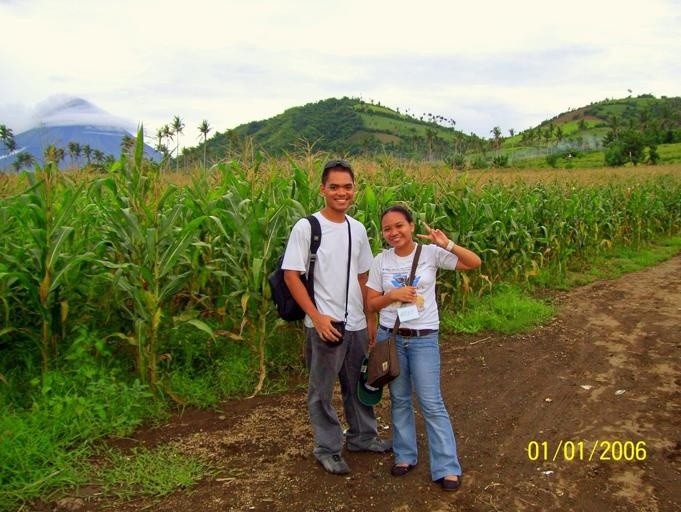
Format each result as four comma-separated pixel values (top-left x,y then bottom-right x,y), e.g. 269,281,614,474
357,358,384,406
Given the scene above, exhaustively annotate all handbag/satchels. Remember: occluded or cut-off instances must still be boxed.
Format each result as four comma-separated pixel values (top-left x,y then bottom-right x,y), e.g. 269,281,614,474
367,335,402,388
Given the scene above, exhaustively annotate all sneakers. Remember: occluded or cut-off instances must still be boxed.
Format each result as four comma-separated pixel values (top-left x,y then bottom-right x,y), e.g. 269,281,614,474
343,435,390,454
312,447,352,477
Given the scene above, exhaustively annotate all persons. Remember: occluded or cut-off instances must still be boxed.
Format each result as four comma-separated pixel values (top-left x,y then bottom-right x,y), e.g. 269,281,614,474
364,205,482,492
281,160,389,475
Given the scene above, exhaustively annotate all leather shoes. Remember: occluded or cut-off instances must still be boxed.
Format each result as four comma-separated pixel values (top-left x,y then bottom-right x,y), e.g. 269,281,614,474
390,457,417,477
441,470,460,492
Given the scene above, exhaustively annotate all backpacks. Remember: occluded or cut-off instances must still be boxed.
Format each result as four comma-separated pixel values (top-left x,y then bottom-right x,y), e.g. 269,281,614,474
267,215,322,323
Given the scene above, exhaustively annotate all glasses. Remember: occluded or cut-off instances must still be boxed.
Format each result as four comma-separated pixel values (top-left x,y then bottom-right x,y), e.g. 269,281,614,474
325,160,352,169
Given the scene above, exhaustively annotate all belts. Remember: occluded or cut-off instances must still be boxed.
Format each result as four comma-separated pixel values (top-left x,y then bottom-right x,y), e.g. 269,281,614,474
380,324,438,337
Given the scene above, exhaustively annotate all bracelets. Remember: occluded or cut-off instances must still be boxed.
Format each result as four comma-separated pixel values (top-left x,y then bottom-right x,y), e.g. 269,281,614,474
445,240,455,251
